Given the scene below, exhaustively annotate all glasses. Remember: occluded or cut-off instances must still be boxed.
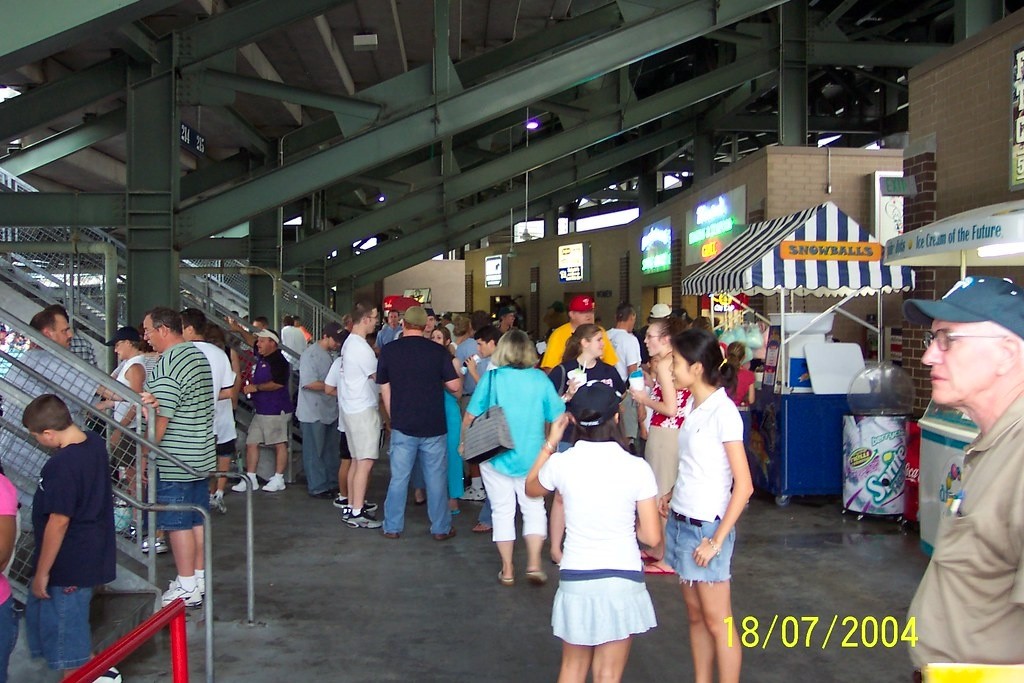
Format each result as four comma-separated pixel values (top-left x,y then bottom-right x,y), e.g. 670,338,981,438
144,324,169,334
923,329,1007,352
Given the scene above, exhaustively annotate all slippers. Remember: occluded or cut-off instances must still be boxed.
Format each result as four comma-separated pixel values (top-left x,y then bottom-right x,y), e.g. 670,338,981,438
640,549,676,574
498,570,549,587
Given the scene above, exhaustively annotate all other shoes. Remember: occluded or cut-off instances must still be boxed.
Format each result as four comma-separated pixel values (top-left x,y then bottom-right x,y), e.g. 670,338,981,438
308,488,340,499
209,495,227,514
378,528,400,539
434,525,457,541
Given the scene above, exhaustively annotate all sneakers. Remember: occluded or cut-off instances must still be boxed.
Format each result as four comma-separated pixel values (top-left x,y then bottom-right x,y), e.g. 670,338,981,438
333,493,382,529
231,476,285,492
162,576,205,607
142,536,169,553
458,486,486,501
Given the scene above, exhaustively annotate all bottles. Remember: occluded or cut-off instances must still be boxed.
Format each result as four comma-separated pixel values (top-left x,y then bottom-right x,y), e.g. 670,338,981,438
111,467,135,534
628,370,644,393
459,353,483,375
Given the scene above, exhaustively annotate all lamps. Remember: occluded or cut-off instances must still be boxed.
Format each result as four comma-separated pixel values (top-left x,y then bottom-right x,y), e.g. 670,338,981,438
506,128,517,258
520,107,533,240
767,312,836,334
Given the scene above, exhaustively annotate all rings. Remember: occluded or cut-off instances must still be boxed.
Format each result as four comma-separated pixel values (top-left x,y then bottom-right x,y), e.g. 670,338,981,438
632,397,634,399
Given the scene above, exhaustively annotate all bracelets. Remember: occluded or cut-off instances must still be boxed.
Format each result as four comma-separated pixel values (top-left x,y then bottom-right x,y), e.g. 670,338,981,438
541,440,556,455
255,384,260,392
708,538,719,555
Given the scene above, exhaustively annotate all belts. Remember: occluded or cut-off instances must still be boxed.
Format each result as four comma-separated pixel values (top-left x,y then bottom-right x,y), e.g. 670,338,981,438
671,511,720,527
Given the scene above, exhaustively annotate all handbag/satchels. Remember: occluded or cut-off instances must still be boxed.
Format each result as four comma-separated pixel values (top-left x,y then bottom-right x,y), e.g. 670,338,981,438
464,369,515,464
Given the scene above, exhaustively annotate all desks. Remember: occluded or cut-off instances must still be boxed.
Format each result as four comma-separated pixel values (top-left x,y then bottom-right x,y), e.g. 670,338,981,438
751,383,900,507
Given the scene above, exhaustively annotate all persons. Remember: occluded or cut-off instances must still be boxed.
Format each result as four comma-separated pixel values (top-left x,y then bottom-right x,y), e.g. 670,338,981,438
905,276,1024,683
0,294,765,683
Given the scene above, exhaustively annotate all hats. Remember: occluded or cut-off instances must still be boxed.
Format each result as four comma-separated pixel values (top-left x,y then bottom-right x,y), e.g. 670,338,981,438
499,306,517,317
650,303,671,318
569,381,622,425
323,321,350,342
568,295,593,312
547,301,564,313
403,306,428,326
902,275,1024,339
252,328,279,344
104,326,140,346
